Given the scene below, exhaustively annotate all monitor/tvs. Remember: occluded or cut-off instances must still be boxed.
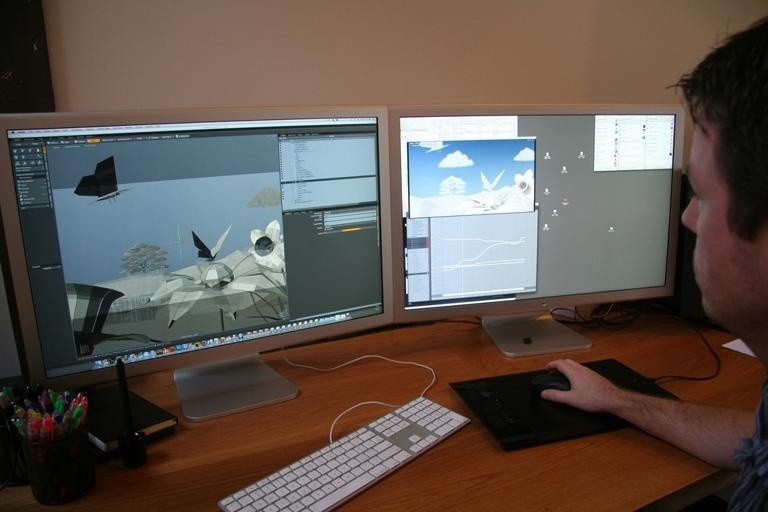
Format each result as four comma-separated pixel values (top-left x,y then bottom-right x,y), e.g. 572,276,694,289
0,106,394,422
387,104,686,358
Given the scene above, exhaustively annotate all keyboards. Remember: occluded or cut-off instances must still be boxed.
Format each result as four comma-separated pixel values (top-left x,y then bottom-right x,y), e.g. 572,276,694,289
217,396,472,512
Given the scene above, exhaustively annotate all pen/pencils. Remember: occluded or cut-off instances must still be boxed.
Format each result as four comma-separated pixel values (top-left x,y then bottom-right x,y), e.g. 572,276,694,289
115,356,139,441
0,381,89,442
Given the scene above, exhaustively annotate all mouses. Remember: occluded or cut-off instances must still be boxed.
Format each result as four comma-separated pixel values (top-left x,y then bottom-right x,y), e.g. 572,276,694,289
530,366,571,392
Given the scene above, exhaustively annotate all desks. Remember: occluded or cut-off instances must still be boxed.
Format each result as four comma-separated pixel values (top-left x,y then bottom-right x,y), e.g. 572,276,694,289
2,319,767,512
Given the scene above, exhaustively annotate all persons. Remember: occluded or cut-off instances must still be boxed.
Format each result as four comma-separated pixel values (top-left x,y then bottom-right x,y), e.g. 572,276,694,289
539,13,768,511
